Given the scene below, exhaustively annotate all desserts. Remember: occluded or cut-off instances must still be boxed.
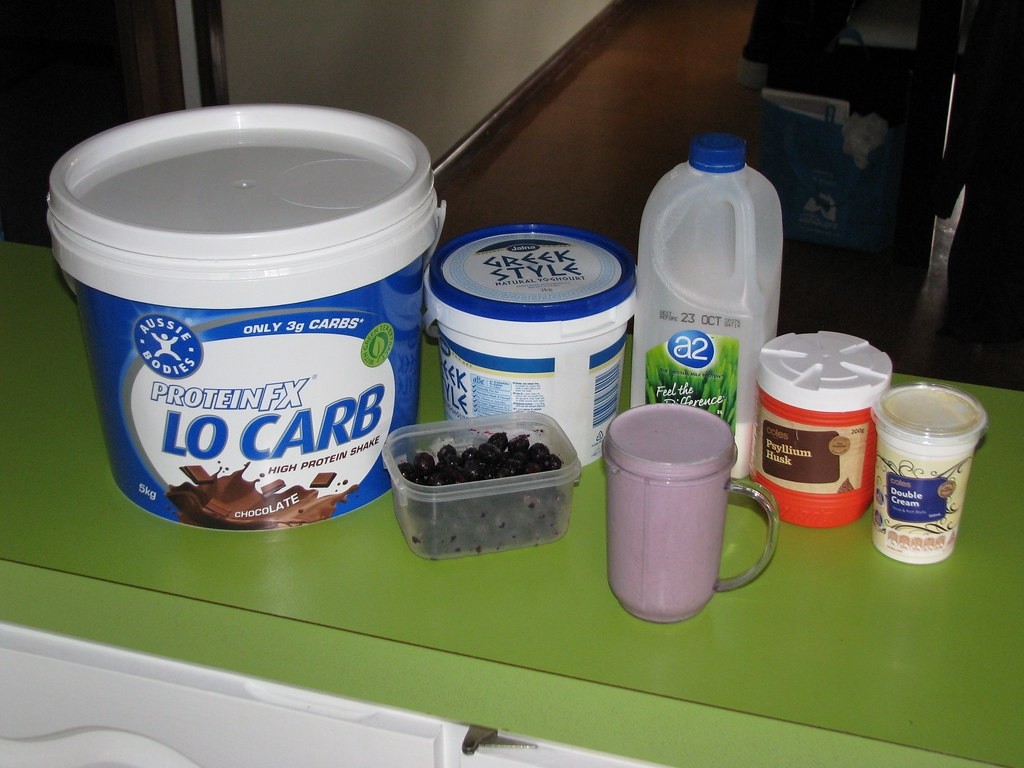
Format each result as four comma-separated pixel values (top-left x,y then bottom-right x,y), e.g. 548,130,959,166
167,465,358,532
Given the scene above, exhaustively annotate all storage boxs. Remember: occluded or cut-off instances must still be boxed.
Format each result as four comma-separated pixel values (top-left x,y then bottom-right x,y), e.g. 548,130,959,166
382,411,581,560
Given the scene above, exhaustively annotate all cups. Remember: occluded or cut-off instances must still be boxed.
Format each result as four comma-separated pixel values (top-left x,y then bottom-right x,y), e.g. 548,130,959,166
747,330,893,528
869,380,988,565
602,403,780,624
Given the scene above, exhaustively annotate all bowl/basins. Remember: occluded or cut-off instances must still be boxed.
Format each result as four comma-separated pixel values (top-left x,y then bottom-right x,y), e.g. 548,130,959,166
381,411,582,560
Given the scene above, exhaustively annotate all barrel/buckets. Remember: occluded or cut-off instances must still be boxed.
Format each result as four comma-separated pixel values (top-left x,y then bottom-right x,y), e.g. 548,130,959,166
420,221,641,468
45,102,447,534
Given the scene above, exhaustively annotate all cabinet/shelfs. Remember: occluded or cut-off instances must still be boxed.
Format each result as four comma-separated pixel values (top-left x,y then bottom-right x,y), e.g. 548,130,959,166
0,242,1024,768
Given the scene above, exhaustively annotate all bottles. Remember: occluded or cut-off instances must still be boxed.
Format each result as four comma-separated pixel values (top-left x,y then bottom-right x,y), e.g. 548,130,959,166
627,131,785,480
